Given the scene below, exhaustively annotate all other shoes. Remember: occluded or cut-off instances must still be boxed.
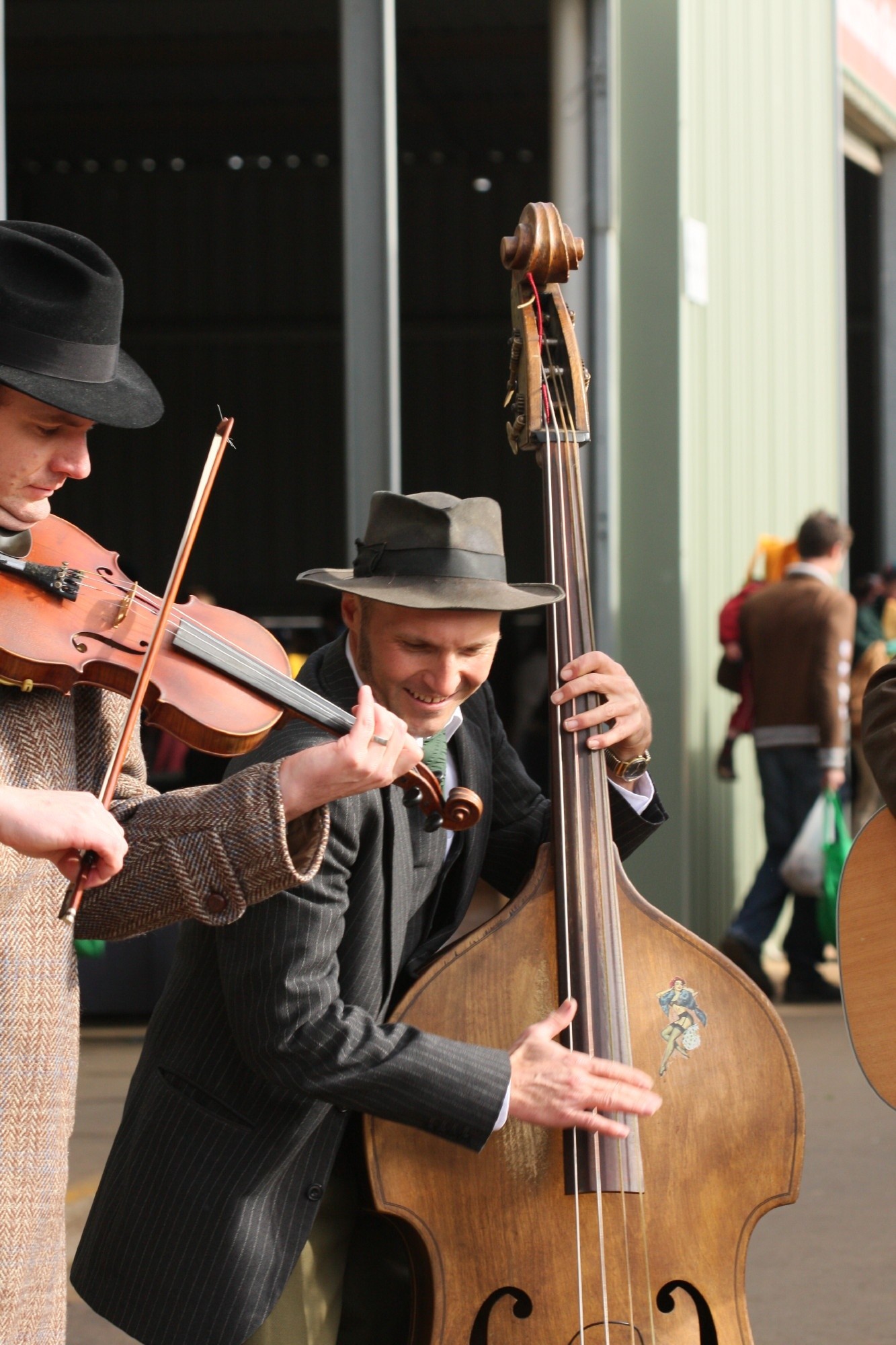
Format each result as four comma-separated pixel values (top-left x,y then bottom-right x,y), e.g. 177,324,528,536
717,932,778,1000
782,967,839,1007
716,752,737,782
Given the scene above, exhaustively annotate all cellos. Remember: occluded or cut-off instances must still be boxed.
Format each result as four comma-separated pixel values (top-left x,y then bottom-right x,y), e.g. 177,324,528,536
359,197,805,1345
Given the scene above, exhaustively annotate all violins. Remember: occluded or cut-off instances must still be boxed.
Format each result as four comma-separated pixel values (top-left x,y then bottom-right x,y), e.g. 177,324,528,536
0,513,485,832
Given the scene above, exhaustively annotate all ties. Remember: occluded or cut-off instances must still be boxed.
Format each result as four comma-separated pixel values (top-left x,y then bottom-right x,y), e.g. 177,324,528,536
421,730,448,795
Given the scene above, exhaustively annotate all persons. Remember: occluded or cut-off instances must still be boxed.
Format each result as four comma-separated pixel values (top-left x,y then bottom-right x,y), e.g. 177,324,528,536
713,511,896,1005
1,219,427,1345
67,488,670,1345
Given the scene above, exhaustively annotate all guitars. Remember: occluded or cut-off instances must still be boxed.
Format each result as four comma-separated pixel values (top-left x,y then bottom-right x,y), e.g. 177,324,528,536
836,804,896,1115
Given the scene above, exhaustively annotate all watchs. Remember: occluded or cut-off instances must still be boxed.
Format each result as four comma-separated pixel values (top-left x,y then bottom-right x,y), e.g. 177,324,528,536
603,747,651,783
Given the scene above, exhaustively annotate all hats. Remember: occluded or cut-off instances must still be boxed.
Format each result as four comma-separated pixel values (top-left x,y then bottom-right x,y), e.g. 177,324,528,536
295,488,567,612
0,221,163,428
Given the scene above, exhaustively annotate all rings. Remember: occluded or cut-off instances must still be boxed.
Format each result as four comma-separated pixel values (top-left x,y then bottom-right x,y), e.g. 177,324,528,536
370,736,388,746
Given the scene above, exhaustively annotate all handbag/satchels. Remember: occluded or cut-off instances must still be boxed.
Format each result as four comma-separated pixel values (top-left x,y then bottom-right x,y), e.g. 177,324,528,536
818,789,853,948
779,789,836,894
